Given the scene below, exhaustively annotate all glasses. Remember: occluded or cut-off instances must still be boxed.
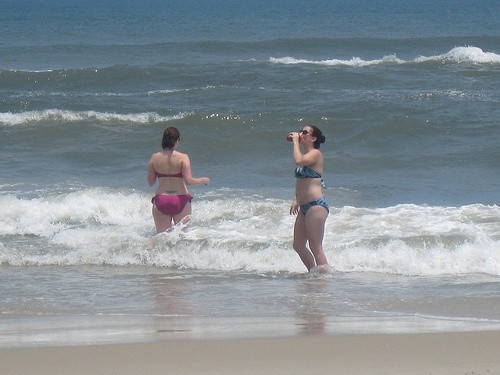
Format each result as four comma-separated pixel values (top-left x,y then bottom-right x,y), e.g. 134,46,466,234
299,130,313,136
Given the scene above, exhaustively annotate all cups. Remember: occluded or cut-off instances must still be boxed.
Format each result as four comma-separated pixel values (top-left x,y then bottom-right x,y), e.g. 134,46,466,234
287,134,301,144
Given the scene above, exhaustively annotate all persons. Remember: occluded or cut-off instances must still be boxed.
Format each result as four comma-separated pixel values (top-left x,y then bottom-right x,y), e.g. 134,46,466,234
288,125,330,274
146,127,210,234
